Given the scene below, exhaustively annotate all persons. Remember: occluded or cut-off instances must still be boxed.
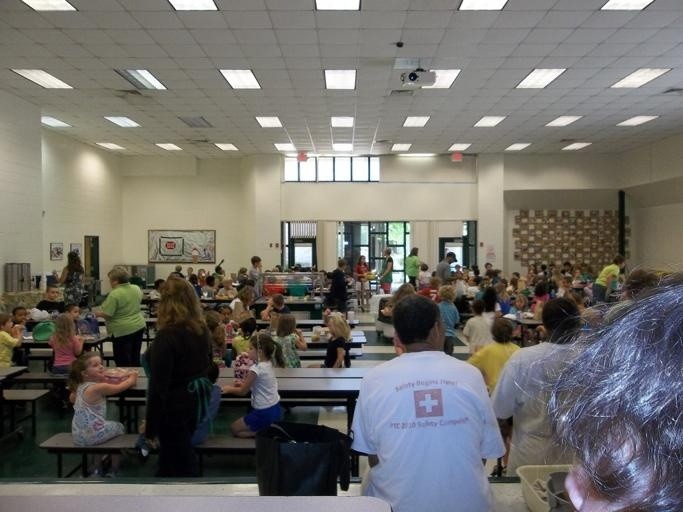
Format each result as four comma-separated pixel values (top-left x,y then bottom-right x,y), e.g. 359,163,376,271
345,295,507,512
0,246,681,479
541,274,681,511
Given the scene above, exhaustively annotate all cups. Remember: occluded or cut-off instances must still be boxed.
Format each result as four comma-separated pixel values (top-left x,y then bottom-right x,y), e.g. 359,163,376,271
347,311,355,323
192,255,198,263
224,348,232,368
312,326,321,336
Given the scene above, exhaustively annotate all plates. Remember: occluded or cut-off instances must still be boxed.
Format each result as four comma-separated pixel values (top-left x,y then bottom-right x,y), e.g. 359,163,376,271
102,372,131,383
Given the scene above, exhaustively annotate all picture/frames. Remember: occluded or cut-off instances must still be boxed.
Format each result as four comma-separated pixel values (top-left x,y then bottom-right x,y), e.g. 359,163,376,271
147,229,216,264
50,242,64,261
70,243,81,258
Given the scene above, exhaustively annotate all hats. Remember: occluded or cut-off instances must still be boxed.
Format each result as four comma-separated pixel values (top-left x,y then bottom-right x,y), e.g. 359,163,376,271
448,252,457,262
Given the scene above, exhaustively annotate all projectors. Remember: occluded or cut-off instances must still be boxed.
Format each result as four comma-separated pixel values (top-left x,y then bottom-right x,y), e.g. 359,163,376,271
400,68,435,86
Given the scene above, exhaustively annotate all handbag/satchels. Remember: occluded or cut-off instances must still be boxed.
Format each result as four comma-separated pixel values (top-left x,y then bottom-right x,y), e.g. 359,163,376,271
32,320,56,340
77,313,100,339
255,422,352,496
